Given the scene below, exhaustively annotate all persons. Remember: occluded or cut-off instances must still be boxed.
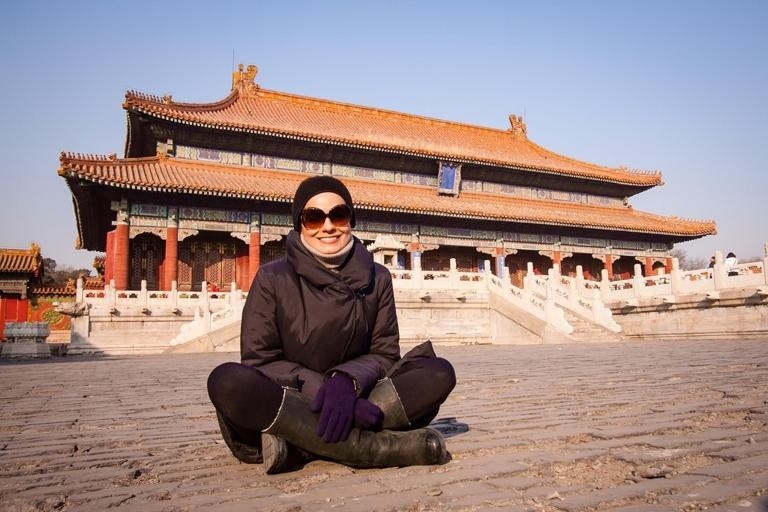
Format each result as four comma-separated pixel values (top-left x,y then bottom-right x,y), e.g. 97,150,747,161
203,174,457,474
724,251,739,277
708,255,715,279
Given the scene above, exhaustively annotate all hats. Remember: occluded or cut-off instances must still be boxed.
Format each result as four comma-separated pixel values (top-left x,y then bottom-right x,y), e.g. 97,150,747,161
292,176,356,232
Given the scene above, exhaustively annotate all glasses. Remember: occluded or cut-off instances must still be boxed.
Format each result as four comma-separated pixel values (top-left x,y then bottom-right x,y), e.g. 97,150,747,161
300,203,353,231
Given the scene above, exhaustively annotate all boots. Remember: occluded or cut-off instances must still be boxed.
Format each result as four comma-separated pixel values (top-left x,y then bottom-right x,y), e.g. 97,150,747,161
260,376,447,474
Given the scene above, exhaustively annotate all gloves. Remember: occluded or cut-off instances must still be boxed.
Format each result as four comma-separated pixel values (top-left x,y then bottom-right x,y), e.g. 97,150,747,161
353,398,382,429
310,372,356,444
